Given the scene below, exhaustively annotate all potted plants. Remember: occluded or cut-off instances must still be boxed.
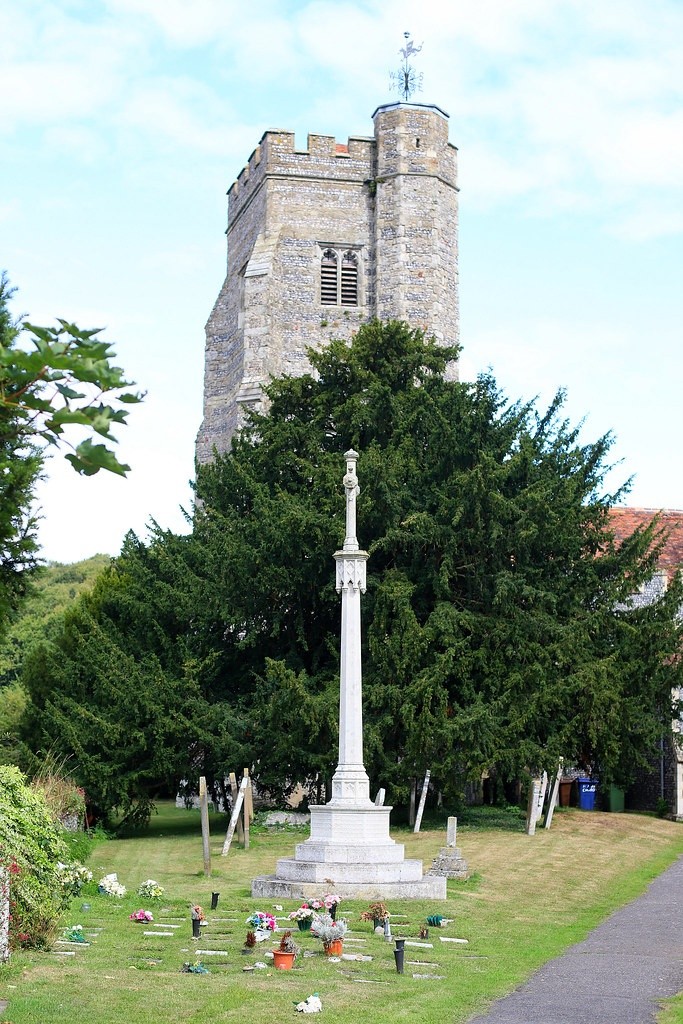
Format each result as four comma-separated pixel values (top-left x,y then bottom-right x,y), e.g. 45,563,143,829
272,930,295,971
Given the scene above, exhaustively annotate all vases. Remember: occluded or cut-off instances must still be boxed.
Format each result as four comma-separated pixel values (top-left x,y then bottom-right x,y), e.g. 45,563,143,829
374,919,384,931
192,919,200,937
393,939,406,974
324,937,344,956
297,919,311,931
328,902,337,921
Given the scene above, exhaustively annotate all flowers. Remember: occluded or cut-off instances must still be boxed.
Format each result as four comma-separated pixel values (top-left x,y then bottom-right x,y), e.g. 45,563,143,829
311,913,350,940
324,892,342,909
290,909,315,921
191,905,205,920
302,898,324,910
362,902,389,921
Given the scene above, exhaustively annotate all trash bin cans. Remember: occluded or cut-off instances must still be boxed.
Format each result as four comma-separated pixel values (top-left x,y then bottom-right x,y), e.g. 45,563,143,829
559,780,578,808
575,777,599,811
600,780,626,813
